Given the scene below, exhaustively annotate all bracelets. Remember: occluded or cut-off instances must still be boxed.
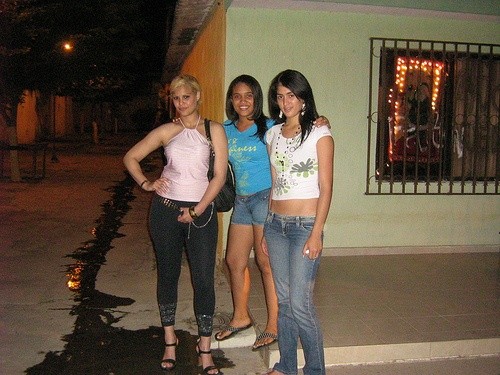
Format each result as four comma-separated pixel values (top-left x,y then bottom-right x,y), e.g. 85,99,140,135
140,180,149,189
188,205,199,220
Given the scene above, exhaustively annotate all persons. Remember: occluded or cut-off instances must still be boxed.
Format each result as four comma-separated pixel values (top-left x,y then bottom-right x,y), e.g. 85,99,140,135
122,73,228,375
265,69,333,375
216,74,332,350
404,81,432,126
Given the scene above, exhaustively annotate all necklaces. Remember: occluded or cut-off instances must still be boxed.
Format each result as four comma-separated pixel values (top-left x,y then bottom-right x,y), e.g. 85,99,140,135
275,123,301,176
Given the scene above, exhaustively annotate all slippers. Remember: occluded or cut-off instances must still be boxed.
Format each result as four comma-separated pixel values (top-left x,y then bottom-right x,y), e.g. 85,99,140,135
252,332,278,352
215,323,252,341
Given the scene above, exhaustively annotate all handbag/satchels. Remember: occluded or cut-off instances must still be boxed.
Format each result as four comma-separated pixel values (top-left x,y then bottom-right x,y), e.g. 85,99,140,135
205,118,236,213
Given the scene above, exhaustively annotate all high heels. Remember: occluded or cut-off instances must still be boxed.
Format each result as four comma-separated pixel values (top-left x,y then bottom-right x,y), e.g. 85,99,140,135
161,337,178,369
196,340,219,375
